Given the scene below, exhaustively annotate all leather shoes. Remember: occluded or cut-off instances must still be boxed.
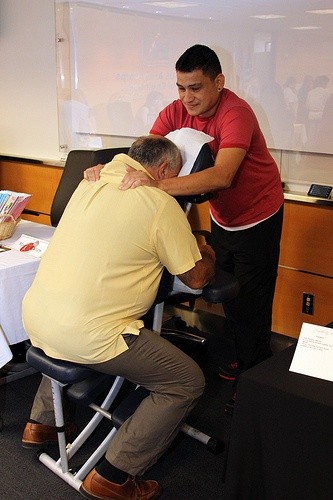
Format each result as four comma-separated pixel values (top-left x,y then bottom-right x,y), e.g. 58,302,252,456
79,467,161,500
21,423,80,449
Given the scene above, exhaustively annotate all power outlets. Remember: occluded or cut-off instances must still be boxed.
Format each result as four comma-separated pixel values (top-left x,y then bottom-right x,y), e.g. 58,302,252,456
302,293,314,315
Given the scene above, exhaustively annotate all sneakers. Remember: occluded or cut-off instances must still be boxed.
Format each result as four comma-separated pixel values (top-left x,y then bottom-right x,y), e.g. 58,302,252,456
218,361,243,381
224,393,236,417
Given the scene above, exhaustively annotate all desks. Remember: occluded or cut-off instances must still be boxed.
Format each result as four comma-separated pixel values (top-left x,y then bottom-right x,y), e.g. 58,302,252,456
223,322,333,500
0,219,61,386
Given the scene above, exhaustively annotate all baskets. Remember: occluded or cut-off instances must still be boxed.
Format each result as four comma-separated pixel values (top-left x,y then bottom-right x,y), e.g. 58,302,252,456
0,214,20,239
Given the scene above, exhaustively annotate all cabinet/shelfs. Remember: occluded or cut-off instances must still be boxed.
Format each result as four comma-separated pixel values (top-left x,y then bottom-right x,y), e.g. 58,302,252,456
0,158,333,344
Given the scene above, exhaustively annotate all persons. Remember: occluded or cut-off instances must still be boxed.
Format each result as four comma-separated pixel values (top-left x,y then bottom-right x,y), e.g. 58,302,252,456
84,44,285,409
22,134,215,500
